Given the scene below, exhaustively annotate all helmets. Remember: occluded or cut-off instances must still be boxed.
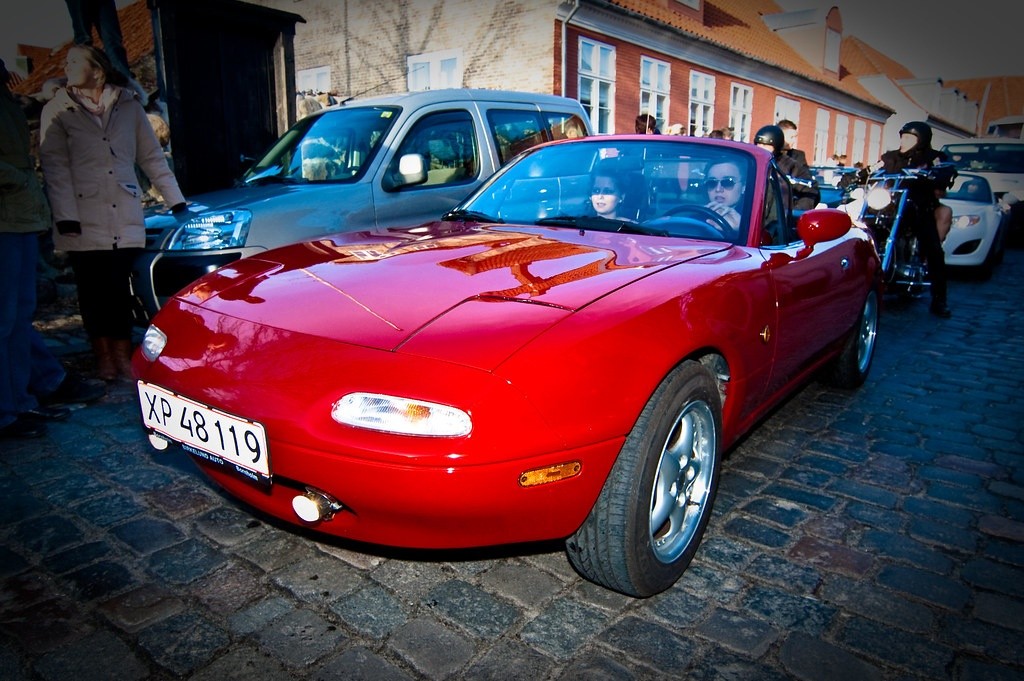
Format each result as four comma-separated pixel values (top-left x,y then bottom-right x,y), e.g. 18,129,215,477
898,120,933,158
752,125,784,156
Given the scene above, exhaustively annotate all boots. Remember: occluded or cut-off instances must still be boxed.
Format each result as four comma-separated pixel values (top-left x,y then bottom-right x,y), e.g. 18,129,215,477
95,335,117,381
113,339,134,378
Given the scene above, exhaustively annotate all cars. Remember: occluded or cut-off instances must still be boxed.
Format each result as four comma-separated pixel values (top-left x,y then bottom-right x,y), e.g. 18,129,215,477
804,164,859,208
935,172,1019,279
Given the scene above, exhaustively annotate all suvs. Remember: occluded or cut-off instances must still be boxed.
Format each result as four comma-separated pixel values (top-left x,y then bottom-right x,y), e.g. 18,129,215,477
937,137,1024,225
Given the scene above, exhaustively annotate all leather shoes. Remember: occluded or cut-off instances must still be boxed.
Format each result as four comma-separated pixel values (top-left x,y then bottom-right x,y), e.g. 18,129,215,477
0,417,49,441
929,302,951,317
37,374,107,407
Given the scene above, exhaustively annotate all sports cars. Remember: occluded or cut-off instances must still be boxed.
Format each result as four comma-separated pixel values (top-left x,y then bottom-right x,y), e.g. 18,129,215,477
130,134,884,600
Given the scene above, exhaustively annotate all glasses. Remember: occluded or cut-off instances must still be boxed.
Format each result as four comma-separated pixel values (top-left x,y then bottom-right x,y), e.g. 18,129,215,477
703,178,743,191
590,187,619,195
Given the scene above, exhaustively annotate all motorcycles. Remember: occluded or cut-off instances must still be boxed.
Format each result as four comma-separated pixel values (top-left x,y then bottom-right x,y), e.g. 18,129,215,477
842,167,932,303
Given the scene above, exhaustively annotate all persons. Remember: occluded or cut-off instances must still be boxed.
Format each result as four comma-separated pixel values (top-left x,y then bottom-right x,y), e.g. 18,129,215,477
928,132,954,243
586,167,644,227
752,124,821,244
1,81,75,436
5,54,174,166
867,120,956,321
295,84,341,118
564,120,578,139
630,103,735,145
773,119,810,173
822,151,886,171
697,158,745,236
40,42,193,384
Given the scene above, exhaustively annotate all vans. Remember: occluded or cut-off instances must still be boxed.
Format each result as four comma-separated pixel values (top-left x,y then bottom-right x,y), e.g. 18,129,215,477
129,87,594,327
